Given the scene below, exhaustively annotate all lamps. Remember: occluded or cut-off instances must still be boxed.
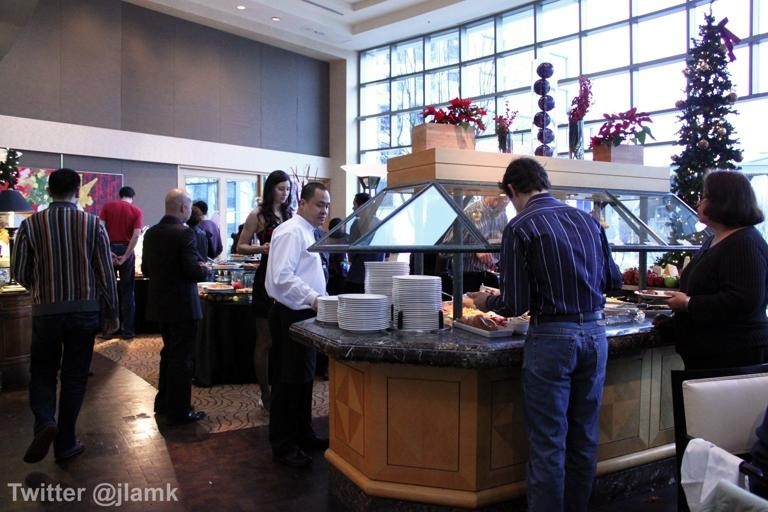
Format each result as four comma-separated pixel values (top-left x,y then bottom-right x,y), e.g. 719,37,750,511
0,190,37,292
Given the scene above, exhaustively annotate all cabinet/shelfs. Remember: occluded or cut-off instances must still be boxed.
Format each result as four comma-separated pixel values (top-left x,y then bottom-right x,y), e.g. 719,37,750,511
1,292,34,391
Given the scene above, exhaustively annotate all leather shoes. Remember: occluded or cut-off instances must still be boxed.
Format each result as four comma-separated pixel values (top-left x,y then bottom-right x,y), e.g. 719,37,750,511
272,442,313,468
167,410,206,426
302,427,325,443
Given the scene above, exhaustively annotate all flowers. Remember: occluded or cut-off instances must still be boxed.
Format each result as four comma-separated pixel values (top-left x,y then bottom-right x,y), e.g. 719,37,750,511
421,98,487,131
568,74,593,121
496,102,518,133
588,108,654,145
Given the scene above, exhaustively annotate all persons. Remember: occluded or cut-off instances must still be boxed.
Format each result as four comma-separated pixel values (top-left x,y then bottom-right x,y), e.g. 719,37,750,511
184,206,209,281
98,186,142,341
9,168,121,466
195,201,223,260
467,157,621,512
236,171,299,414
651,170,767,512
141,189,211,430
263,181,329,471
231,194,511,300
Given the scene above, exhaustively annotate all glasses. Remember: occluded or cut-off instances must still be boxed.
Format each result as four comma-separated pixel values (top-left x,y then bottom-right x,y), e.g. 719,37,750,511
698,194,706,204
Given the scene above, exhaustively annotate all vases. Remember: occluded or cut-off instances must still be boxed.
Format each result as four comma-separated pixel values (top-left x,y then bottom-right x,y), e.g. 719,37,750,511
412,123,477,152
498,129,512,152
569,121,584,159
593,144,644,164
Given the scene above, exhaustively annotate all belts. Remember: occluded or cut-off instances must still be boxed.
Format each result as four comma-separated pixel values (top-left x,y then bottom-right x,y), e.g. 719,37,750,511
530,311,605,324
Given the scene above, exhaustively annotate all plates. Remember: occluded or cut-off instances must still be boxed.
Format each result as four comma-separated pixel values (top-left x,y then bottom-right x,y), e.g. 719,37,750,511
210,261,239,269
316,260,443,337
196,281,234,291
636,288,674,300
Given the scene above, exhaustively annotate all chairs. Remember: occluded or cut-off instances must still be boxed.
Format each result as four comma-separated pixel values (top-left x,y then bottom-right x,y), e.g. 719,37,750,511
669,366,768,511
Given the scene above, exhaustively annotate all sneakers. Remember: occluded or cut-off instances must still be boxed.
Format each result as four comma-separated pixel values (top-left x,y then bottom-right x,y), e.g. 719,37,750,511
24,424,57,463
54,439,85,461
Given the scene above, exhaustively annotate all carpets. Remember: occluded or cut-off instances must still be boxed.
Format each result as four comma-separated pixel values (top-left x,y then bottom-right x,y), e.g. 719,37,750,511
91,335,330,434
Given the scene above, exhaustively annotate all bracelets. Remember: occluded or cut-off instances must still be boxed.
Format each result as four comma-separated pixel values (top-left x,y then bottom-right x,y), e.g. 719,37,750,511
684,296,693,308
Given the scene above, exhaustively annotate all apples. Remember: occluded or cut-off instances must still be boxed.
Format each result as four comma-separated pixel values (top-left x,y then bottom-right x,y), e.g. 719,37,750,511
623,270,681,288
232,282,241,289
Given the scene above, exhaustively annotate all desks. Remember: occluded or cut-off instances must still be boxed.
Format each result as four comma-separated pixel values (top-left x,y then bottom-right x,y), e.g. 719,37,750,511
195,293,257,387
290,297,684,508
